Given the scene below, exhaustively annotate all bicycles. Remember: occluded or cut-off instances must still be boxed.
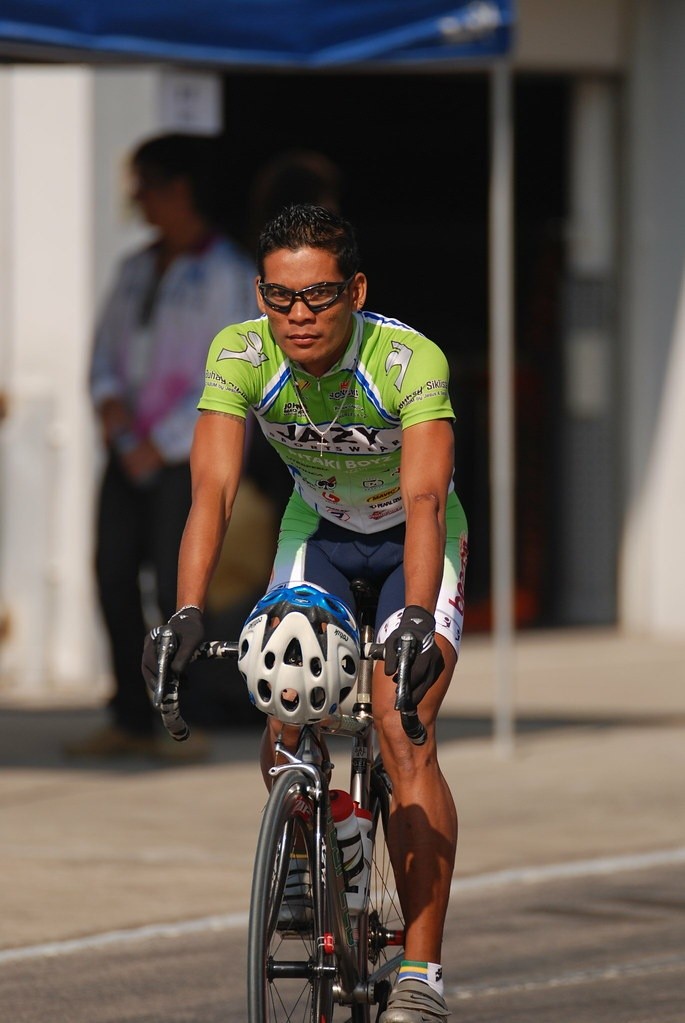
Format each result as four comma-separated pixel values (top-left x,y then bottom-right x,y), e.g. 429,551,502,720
151,628,427,1022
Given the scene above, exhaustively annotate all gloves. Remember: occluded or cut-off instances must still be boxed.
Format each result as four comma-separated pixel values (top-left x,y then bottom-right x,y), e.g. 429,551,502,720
384,605,445,711
142,605,205,708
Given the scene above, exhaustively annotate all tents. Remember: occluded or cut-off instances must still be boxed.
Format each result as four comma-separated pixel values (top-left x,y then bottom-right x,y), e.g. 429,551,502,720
0,1,517,770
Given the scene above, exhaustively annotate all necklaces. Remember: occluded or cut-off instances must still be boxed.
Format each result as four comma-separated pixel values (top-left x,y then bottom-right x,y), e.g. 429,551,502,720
289,363,355,461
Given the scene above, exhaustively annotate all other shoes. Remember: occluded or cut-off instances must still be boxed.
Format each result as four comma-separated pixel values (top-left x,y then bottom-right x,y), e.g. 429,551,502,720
276,858,316,936
379,976,452,1023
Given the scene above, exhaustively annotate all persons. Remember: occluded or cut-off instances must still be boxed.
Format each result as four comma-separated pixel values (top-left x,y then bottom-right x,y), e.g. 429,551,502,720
142,205,470,1022
68,129,264,763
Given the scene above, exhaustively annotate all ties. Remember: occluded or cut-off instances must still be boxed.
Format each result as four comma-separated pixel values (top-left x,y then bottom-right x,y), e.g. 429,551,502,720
139,259,168,329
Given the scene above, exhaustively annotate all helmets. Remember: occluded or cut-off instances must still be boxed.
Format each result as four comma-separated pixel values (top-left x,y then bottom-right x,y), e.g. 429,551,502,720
237,581,360,726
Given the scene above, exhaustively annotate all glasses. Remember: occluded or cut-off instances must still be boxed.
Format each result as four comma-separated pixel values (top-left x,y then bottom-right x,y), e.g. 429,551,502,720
259,273,356,312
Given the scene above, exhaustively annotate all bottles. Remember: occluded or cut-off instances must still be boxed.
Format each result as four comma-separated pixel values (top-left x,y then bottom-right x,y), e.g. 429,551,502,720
329,789,364,878
344,801,374,915
